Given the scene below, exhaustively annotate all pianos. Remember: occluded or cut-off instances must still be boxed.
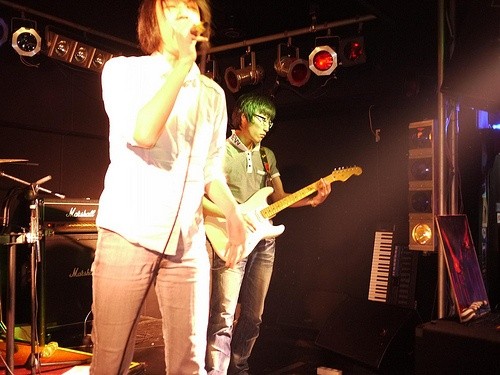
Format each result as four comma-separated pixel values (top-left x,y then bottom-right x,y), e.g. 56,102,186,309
367,222,399,305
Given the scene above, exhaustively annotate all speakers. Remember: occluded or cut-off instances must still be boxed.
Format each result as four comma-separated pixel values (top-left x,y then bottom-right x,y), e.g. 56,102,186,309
35,232,98,348
314,296,422,369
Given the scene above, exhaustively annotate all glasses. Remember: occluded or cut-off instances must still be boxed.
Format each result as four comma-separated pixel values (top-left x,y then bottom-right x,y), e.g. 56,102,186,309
254,114,273,129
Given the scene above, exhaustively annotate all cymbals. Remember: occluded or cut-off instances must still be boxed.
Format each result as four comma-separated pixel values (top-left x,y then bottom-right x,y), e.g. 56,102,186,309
0,158,29,164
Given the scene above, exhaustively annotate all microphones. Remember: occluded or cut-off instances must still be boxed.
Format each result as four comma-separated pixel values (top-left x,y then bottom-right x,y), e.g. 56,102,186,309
190,21,209,36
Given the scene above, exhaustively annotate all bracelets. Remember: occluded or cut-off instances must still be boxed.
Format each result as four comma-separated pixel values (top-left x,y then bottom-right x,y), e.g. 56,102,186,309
308,195,318,208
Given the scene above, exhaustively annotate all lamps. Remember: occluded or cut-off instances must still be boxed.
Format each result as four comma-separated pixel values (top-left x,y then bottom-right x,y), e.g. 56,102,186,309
273,37,311,86
330,24,366,67
309,28,338,76
45,25,123,74
224,45,265,93
408,120,437,250
10,11,41,57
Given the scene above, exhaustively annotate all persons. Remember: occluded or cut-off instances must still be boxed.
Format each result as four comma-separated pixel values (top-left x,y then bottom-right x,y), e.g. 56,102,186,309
89,0,248,375
202,92,332,375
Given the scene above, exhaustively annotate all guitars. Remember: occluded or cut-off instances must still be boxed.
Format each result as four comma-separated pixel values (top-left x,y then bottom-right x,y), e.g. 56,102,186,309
204,164,363,261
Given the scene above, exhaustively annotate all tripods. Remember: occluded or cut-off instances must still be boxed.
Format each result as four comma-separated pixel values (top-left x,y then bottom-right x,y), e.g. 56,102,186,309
0,173,95,375
